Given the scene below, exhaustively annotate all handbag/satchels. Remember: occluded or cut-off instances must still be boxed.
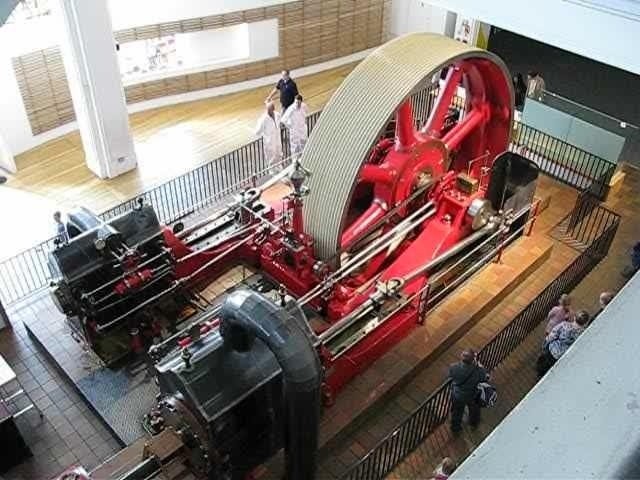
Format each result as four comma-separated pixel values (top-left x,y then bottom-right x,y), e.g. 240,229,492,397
472,366,497,408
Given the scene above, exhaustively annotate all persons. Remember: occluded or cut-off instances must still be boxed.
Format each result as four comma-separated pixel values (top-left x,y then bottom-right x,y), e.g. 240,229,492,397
53,211,65,234
544,294,574,336
448,348,497,433
264,68,298,117
536,310,590,381
511,71,527,106
257,102,283,176
621,241,640,277
433,457,457,480
280,94,309,160
526,69,544,97
600,292,613,309
